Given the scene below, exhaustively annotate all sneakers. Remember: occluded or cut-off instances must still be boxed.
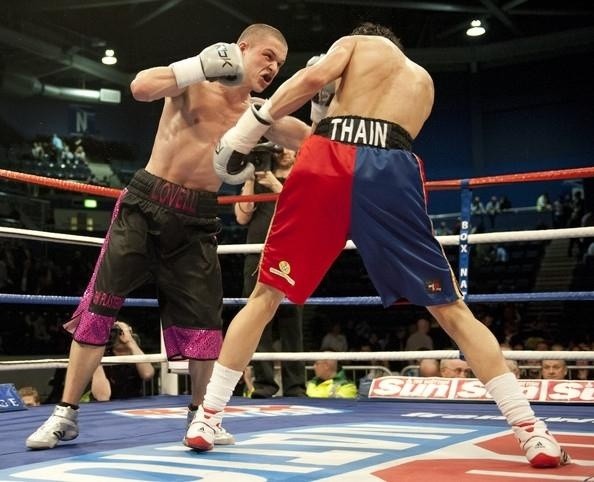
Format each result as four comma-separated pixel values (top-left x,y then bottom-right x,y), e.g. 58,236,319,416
510,418,572,468
181,404,236,451
24,405,80,452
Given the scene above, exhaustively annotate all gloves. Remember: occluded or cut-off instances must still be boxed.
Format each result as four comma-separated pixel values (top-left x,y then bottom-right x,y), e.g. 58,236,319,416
170,41,244,88
306,53,336,125
211,101,275,186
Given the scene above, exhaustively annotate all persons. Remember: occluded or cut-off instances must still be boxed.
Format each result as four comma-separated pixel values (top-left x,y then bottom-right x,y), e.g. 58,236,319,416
24,22,311,450
182,22,572,470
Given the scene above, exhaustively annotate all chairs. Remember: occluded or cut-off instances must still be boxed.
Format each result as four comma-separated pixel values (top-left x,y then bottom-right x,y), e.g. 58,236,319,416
0,151,93,193
333,219,551,355
